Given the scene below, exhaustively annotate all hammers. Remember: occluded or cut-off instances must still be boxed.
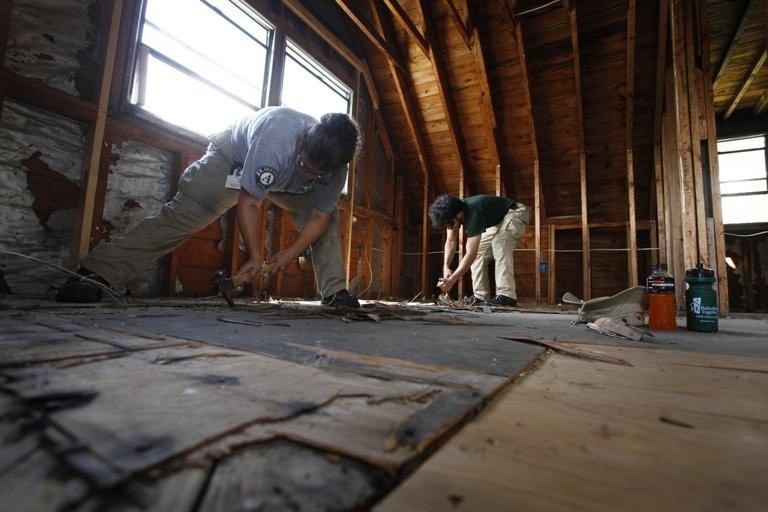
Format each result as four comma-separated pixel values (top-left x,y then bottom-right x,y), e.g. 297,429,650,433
214,263,275,306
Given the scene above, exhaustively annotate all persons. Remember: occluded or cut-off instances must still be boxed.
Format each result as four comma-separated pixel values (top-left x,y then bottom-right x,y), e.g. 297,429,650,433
429,194,532,307
54,105,360,308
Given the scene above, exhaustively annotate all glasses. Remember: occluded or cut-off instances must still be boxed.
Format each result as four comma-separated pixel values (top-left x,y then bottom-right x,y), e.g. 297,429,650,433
296,154,333,185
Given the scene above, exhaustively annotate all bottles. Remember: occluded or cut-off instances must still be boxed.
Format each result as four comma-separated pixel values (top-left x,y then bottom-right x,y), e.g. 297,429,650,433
683,262,719,333
645,263,678,331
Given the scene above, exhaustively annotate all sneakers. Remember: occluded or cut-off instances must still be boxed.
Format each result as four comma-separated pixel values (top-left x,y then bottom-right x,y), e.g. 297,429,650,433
460,296,483,306
321,289,360,308
484,295,517,307
56,267,103,303
